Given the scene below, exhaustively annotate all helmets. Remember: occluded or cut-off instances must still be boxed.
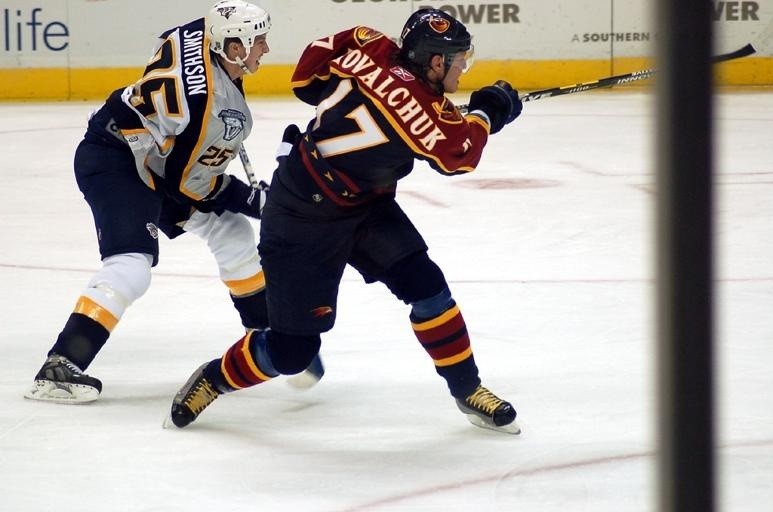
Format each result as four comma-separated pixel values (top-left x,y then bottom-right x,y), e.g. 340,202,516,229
396,9,475,75
208,0,272,65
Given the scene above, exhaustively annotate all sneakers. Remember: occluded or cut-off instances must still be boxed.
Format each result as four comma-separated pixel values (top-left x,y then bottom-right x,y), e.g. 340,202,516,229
455,385,516,427
35,353,102,394
172,358,236,428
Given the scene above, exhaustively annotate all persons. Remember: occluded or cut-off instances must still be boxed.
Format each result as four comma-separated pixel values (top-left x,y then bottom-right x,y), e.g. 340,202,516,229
19,2,269,402
167,4,525,427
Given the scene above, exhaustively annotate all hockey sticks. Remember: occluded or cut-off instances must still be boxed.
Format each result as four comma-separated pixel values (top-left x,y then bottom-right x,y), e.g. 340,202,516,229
238,145,325,389
454,20,772,112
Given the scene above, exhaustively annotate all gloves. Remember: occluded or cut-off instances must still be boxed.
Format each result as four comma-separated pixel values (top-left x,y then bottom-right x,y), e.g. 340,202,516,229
468,81,523,134
214,175,270,219
